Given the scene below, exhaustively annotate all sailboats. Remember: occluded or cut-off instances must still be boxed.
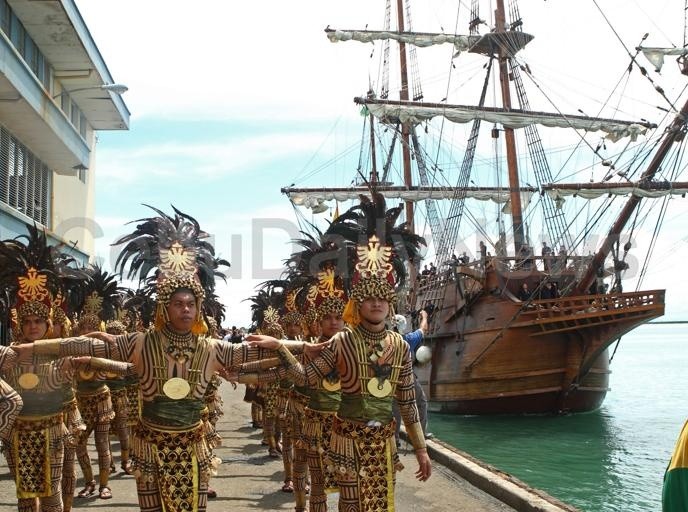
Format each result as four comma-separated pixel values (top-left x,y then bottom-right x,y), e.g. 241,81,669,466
280,1,688,419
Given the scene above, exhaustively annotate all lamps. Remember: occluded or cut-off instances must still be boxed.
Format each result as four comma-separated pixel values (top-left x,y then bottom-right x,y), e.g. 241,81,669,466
49,82,129,103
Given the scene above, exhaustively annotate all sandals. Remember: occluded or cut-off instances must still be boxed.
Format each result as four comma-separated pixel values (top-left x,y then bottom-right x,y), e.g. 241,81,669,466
121,461,134,474
99,486,111,498
78,482,94,497
253,421,311,493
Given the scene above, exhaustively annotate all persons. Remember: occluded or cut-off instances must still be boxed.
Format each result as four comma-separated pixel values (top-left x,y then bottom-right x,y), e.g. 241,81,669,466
428,262,437,276
557,245,568,271
519,283,532,301
488,252,491,256
539,281,557,298
551,251,557,265
0,288,432,512
457,252,470,264
540,241,550,270
421,264,430,276
476,241,487,258
441,253,459,267
519,244,532,271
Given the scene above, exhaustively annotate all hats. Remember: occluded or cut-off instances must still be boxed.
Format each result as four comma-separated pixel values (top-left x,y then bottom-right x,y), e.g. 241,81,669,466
258,261,398,335
155,270,203,313
15,291,126,334
